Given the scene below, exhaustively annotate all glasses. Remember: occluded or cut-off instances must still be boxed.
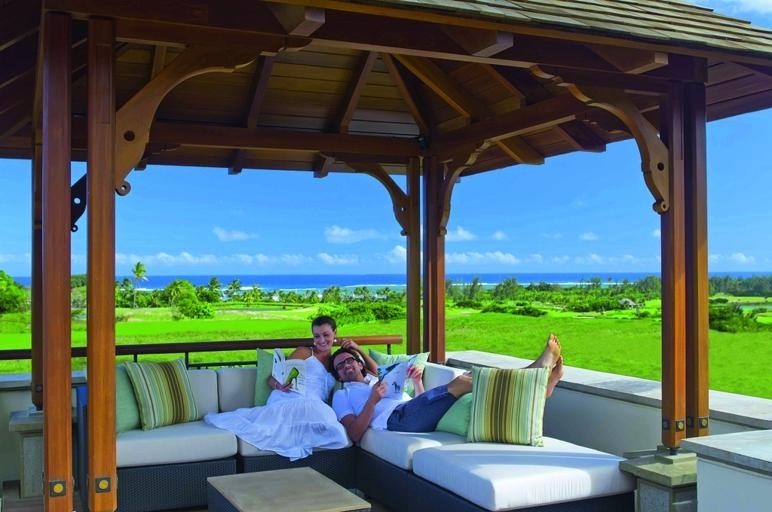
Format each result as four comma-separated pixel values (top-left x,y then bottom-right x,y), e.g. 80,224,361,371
336,357,359,371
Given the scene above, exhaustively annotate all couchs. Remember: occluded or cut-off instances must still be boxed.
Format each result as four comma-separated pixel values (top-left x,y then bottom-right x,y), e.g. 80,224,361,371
112,367,636,512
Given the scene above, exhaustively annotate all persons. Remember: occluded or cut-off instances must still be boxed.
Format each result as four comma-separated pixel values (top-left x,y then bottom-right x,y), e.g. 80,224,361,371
267,316,563,442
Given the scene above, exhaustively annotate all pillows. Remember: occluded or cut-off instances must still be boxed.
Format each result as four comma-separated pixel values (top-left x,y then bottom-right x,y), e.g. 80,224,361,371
368,349,431,398
253,346,275,407
433,364,552,448
115,359,200,434
